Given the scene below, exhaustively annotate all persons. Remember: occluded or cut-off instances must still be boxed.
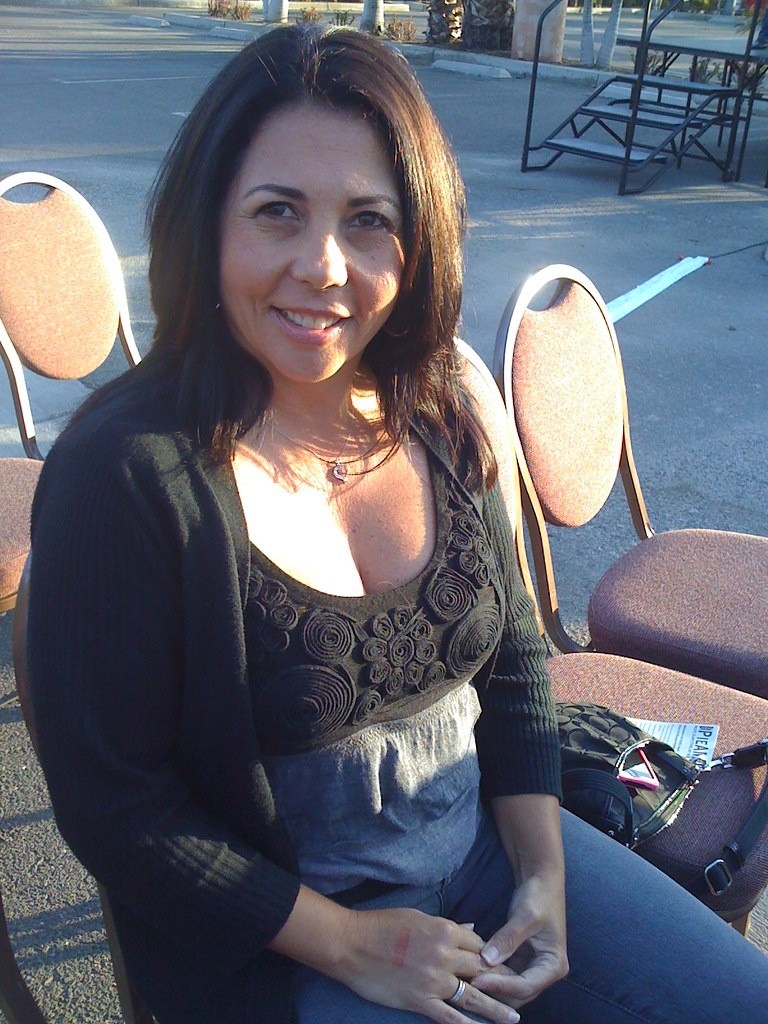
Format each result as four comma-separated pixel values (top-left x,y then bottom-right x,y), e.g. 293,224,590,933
30,22,768,1024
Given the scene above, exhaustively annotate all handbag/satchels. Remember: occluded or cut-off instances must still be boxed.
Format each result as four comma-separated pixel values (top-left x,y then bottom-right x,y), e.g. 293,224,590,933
555,700,768,900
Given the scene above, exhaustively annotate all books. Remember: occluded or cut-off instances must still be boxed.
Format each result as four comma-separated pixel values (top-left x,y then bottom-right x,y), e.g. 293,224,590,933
624,716,719,766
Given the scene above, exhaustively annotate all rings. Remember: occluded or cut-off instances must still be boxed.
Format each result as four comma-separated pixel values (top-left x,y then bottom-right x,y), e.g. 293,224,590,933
450,979,465,1003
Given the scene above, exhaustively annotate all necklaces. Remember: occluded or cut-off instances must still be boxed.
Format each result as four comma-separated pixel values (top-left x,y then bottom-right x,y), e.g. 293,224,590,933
258,369,356,483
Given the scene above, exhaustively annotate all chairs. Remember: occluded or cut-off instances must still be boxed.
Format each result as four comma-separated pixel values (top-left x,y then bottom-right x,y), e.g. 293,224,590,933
0,171,142,615
492,262,768,700
452,332,768,937
